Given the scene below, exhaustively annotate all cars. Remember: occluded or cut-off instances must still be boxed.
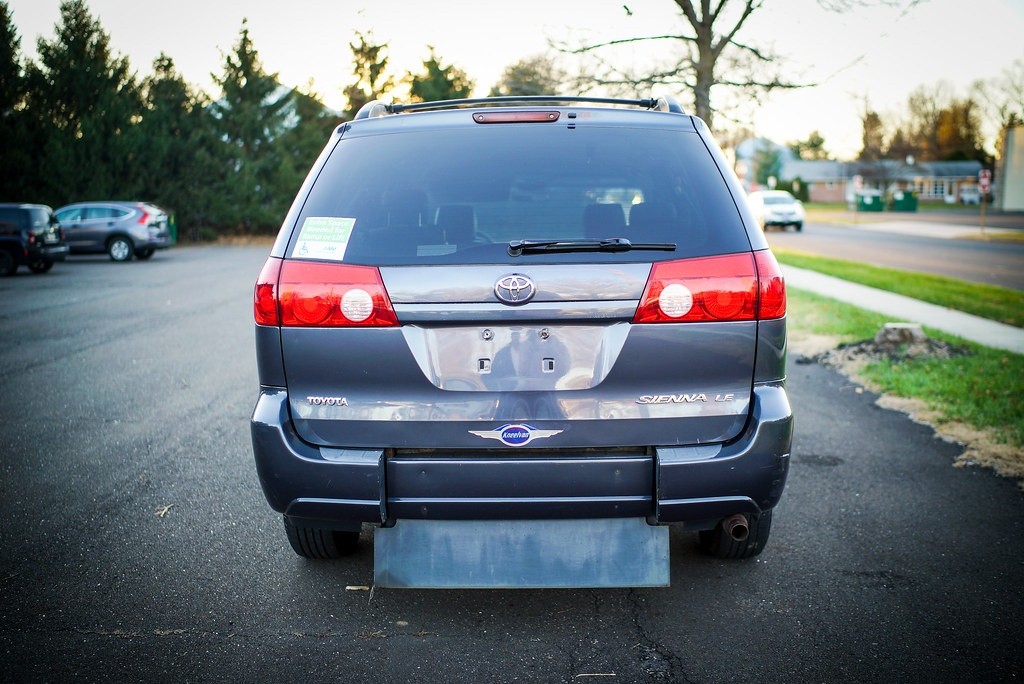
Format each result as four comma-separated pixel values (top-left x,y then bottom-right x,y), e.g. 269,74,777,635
749,188,804,233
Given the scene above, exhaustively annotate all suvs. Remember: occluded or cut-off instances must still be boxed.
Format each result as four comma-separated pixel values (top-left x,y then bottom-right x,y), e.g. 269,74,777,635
246,95,795,587
53,200,176,262
0,201,69,278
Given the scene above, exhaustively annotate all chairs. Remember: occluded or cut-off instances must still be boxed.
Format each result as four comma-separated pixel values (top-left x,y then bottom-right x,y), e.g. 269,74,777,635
582,202,669,237
362,188,476,253
